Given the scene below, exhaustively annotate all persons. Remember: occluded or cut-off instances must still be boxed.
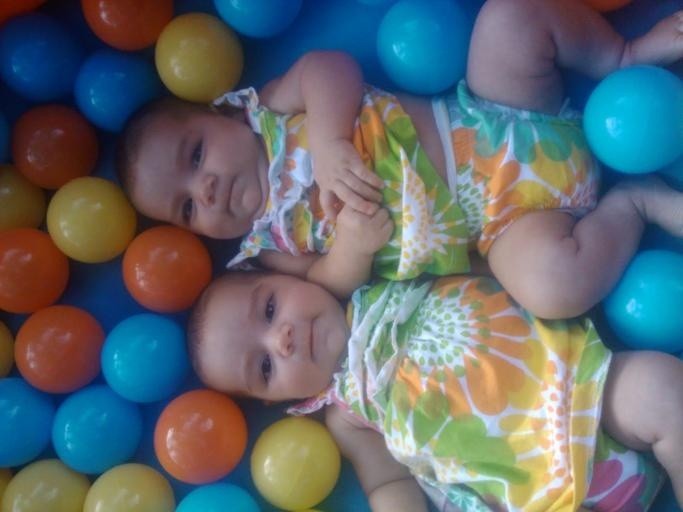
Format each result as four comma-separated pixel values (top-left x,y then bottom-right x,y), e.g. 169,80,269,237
185,268,683,511
111,1,683,323
